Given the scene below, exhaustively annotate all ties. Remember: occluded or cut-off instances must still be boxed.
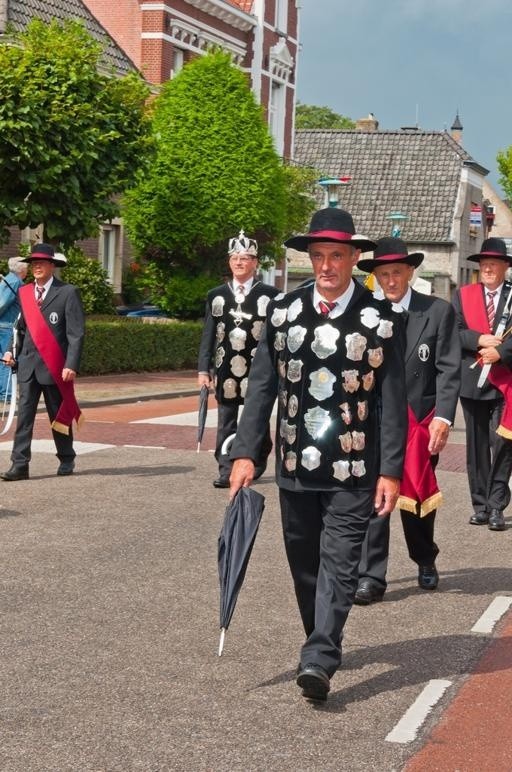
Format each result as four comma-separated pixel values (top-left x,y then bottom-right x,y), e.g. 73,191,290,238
487,291,498,331
238,285,245,297
319,301,337,321
36,287,45,310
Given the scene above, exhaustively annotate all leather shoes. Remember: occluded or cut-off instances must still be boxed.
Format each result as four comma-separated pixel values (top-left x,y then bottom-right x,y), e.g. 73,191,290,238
214,475,231,489
354,582,387,607
297,663,331,703
418,564,440,591
57,460,75,476
488,509,506,531
469,511,491,526
1,467,31,481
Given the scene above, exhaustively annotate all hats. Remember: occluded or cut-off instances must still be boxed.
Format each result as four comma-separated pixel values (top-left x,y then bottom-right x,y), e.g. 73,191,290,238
284,207,379,254
228,230,259,257
357,237,424,274
467,238,512,267
18,244,67,267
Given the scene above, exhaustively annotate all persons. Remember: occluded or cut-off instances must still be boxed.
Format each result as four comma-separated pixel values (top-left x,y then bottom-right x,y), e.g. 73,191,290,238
227,206,407,702
0,256,30,403
453,236,512,531
0,242,81,481
197,230,274,487
354,236,461,606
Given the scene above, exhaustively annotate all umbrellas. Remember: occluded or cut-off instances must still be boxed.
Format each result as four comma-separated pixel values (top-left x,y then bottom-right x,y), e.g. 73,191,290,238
216,485,265,657
196,373,209,452
